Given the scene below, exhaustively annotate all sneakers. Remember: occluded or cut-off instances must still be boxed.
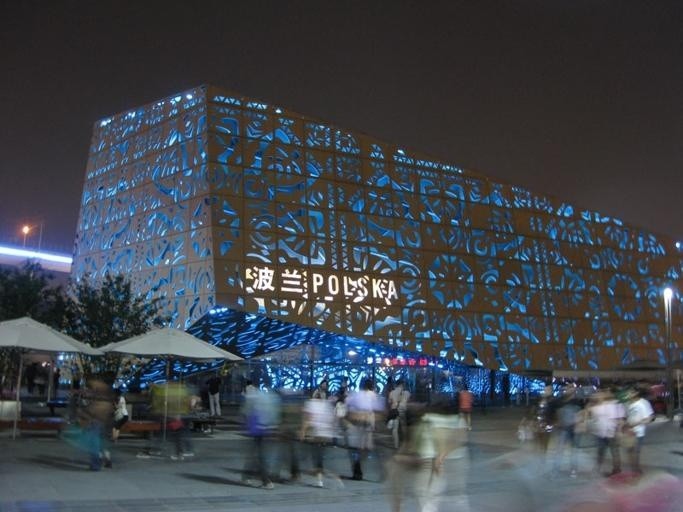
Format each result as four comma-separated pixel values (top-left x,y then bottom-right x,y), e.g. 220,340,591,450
257,484,274,490
311,481,324,487
352,469,364,480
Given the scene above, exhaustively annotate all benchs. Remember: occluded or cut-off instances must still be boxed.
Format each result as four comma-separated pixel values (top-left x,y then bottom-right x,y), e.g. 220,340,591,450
0,418,164,454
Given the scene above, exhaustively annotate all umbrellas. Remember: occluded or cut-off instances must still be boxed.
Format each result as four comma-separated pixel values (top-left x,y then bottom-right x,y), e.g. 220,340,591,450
0,312,105,438
98,316,244,442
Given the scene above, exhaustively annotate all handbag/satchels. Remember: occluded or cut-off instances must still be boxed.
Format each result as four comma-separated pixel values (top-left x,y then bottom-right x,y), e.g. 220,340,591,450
386,407,400,419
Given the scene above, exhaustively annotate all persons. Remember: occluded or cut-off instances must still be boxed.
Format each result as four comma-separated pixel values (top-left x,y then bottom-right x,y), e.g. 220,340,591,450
206,372,221,416
520,381,667,483
238,372,473,511
77,373,112,471
110,388,128,442
135,379,194,461
20,357,63,399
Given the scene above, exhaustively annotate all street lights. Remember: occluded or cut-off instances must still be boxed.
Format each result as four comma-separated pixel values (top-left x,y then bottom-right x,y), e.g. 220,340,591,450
664,287,673,419
